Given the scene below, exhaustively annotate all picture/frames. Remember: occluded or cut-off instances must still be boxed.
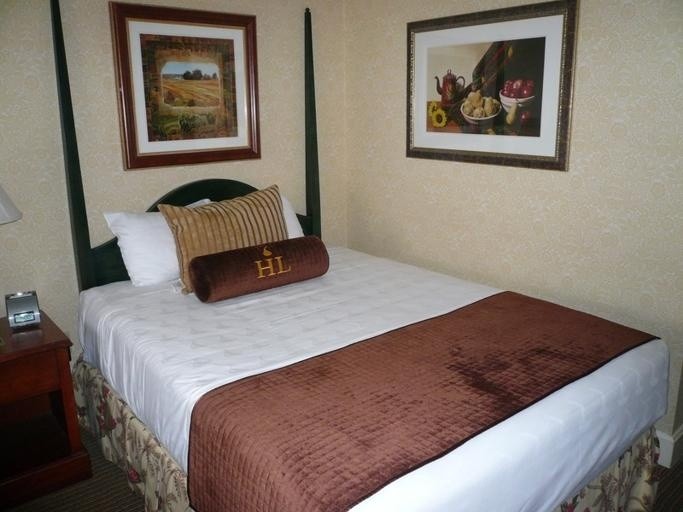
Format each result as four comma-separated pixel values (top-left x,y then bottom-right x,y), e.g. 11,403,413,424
107,0,264,171
401,0,580,172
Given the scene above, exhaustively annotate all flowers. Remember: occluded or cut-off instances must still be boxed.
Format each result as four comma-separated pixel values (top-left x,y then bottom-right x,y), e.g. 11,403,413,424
426,97,464,128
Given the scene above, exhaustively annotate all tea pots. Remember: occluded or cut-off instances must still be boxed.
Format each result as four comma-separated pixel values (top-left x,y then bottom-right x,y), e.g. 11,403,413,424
434,68,465,111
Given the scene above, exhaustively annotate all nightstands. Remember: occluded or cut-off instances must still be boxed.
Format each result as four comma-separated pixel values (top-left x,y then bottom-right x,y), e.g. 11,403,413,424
0,305,93,503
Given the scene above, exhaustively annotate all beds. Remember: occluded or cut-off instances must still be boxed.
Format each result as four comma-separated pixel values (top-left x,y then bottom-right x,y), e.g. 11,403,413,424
50,2,673,511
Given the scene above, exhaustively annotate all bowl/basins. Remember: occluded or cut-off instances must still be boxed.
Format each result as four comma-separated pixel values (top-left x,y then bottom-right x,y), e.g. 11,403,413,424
459,96,501,127
498,88,536,114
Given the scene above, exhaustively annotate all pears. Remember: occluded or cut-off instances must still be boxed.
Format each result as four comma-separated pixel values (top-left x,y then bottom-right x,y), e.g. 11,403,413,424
463,91,498,118
506,102,519,125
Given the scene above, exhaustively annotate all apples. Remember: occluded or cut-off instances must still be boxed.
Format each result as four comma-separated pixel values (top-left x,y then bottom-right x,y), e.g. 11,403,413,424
502,80,535,98
519,111,533,125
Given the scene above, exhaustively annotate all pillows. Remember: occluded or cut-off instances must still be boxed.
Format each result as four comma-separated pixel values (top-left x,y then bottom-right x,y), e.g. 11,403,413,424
99,196,211,290
186,233,330,304
155,180,290,295
276,191,306,240
